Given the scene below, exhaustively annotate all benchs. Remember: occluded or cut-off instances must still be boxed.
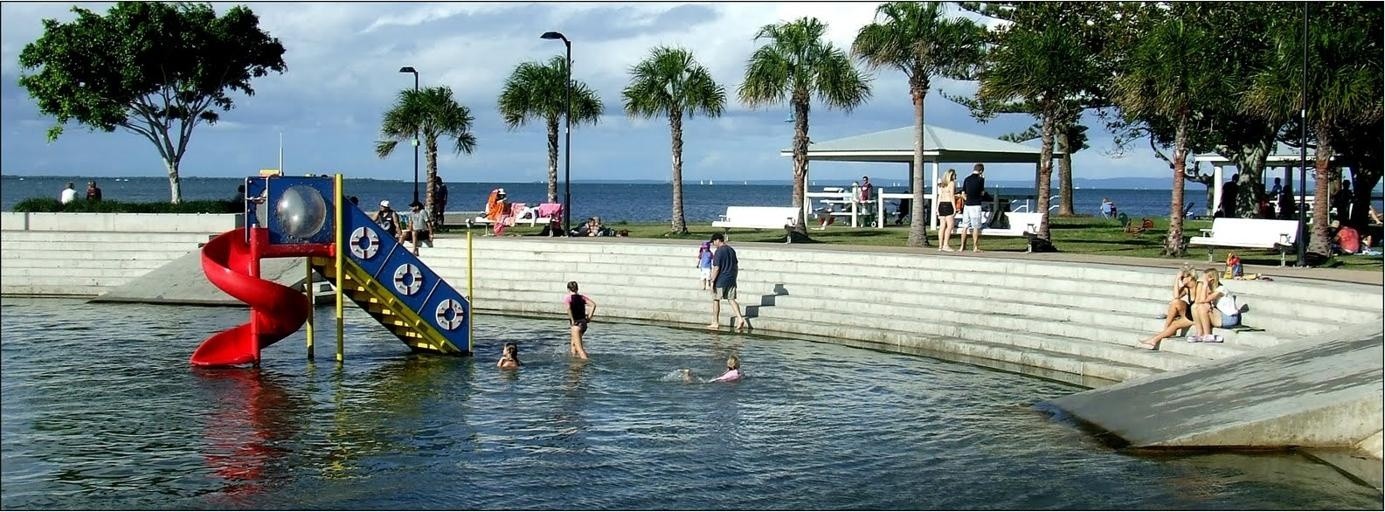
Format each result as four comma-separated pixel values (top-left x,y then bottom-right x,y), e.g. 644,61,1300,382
829,212,872,216
1269,194,1338,225
711,205,806,245
475,201,564,239
1189,217,1300,270
936,212,1045,254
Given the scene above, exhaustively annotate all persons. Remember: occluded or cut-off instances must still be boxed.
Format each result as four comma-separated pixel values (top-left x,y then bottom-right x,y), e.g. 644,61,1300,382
86,181,102,201
571,218,595,236
1100,198,1113,216
398,200,433,255
710,354,745,382
895,191,908,224
697,241,713,290
937,168,957,252
1138,253,1249,346
1327,179,1383,255
61,183,75,204
233,185,245,202
588,216,606,237
707,232,745,329
1217,173,1295,219
372,199,402,238
497,188,509,213
835,175,873,225
436,176,448,232
497,343,519,368
958,164,986,254
562,281,597,359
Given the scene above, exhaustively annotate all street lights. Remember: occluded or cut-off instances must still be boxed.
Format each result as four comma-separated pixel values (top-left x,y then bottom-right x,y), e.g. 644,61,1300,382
398,67,419,201
538,30,572,237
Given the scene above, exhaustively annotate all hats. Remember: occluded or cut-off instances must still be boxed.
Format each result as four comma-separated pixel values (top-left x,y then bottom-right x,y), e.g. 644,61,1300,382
499,188,505,194
709,232,723,243
701,242,710,249
409,201,420,206
380,200,390,208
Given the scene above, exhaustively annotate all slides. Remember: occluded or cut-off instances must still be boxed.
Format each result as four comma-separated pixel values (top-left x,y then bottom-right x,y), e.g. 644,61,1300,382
189,228,308,366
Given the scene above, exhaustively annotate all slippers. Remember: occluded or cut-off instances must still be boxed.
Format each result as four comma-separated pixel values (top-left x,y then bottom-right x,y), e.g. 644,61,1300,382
1201,334,1224,343
1186,335,1202,343
1156,314,1166,319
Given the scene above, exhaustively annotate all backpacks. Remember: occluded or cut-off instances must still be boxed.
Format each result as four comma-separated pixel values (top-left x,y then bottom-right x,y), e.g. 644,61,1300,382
985,207,1009,228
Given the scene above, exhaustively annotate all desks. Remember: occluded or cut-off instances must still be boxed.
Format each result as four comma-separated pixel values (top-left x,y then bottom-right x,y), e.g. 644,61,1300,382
820,198,876,228
